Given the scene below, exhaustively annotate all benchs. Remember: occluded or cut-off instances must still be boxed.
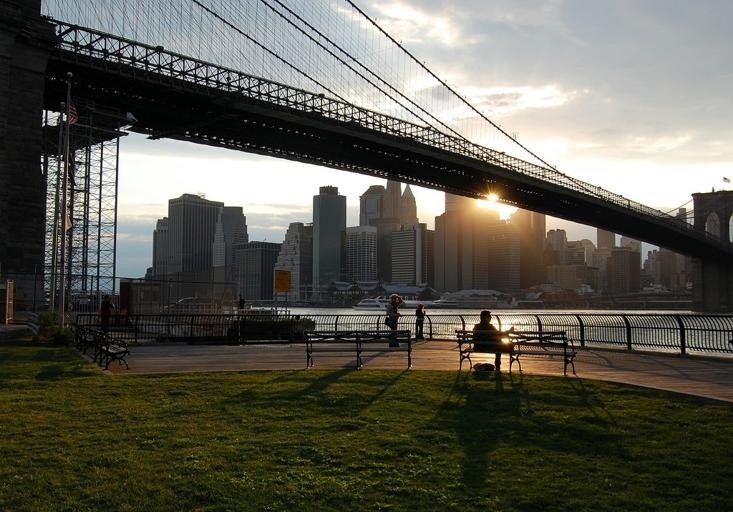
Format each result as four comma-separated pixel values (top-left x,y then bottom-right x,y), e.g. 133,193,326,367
75,328,131,370
455,328,578,376
303,329,413,370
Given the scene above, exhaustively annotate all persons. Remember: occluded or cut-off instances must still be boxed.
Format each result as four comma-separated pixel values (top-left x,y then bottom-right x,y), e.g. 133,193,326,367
386,293,403,351
100,294,114,333
232,296,239,319
237,295,246,320
472,311,514,375
415,303,425,340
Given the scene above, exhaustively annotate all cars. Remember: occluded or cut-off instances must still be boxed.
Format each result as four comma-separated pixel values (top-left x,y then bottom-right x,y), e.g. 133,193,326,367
161,297,221,309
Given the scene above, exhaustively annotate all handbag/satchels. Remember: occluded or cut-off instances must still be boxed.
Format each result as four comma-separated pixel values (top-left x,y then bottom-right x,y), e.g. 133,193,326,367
385,316,397,330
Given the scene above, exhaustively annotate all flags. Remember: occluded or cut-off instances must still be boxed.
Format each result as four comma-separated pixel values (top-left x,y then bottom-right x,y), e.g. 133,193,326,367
723,177,731,183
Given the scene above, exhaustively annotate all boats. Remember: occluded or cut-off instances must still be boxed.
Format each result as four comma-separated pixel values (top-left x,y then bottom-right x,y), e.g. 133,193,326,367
352,298,389,311
